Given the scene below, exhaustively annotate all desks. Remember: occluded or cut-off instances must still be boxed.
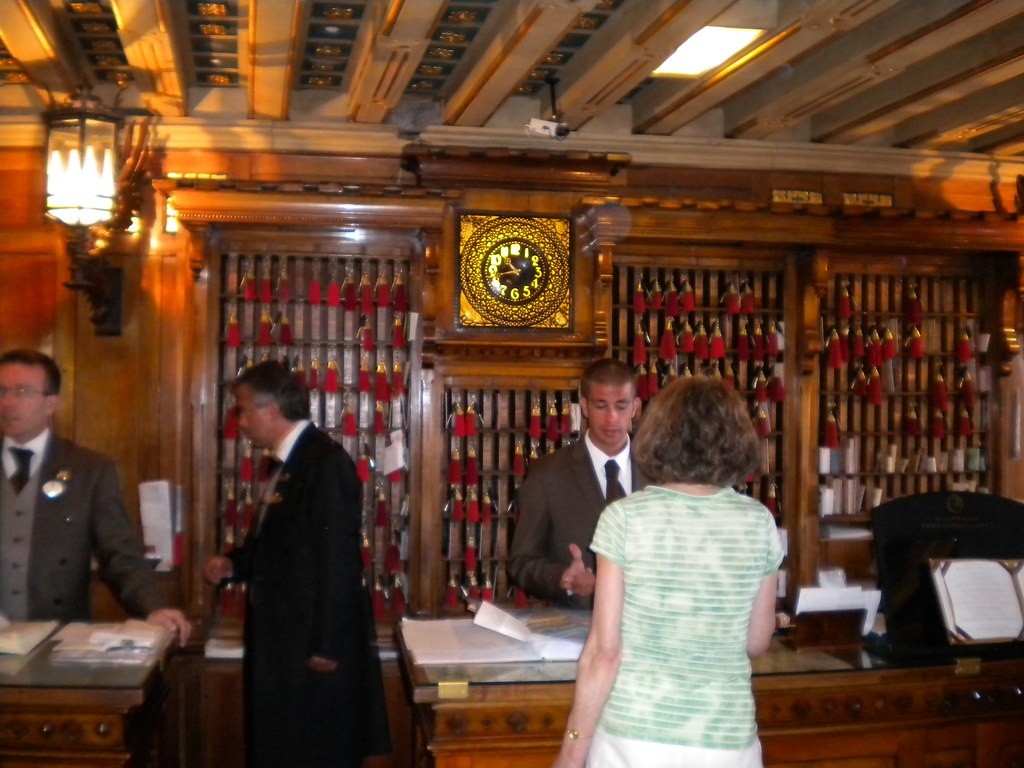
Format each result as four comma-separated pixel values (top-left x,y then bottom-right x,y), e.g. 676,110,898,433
395,614,1023,768
0,620,181,768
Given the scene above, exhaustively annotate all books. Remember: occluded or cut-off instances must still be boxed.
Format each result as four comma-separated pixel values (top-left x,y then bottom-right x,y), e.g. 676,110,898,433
0,618,170,657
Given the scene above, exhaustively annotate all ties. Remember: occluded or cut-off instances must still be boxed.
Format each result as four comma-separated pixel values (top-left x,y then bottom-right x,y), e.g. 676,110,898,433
8,447,34,494
604,461,628,504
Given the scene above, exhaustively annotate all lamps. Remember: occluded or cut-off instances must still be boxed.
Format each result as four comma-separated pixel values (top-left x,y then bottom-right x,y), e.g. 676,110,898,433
38,81,135,294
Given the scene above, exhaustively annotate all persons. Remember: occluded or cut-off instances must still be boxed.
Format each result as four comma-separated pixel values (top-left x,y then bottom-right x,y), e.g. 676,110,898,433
205,359,395,767
547,377,787,768
506,358,654,610
2,351,192,648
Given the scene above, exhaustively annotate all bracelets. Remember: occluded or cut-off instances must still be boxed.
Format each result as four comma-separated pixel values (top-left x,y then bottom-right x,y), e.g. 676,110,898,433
566,727,595,740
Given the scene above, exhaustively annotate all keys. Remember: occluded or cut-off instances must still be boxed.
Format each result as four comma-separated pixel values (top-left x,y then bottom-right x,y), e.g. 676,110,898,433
614,259,988,529
214,258,584,632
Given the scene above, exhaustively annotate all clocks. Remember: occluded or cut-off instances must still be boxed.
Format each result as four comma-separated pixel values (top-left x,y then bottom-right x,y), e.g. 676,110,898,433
458,212,577,332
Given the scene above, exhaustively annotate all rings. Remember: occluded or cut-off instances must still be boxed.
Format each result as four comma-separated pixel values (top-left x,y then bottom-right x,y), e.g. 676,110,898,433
563,577,574,584
